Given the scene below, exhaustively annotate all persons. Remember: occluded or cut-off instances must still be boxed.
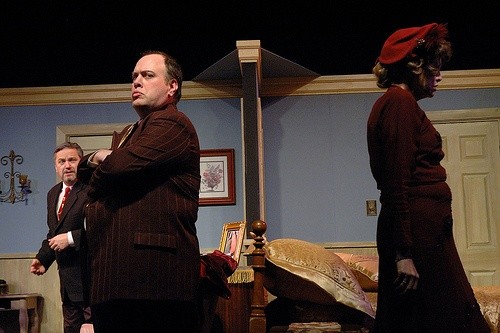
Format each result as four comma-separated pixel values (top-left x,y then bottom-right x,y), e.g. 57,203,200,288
224,232,237,258
367,24,493,333
31,141,84,333
77,51,201,333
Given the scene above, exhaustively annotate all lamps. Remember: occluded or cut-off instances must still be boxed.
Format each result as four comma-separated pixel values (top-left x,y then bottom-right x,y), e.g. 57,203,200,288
0,150,32,206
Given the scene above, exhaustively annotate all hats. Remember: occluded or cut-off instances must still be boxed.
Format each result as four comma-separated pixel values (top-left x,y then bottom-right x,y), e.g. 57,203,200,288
379,22,437,65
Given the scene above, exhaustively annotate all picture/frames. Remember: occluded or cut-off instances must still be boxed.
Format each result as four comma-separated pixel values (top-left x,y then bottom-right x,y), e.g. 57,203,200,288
219,222,245,264
197,148,236,205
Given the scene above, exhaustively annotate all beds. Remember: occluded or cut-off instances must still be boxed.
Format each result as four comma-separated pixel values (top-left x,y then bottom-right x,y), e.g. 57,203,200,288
247,220,500,333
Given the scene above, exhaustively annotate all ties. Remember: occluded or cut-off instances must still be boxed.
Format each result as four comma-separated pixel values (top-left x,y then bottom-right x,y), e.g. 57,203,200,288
58,187,71,221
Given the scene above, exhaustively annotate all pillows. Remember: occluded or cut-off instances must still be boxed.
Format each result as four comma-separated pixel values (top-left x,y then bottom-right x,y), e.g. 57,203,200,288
334,252,379,290
243,232,375,318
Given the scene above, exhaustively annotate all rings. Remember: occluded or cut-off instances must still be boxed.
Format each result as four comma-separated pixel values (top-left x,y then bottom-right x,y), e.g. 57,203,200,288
403,280,408,285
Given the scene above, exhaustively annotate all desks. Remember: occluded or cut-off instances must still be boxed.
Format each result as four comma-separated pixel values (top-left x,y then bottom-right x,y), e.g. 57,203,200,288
0,293,42,333
